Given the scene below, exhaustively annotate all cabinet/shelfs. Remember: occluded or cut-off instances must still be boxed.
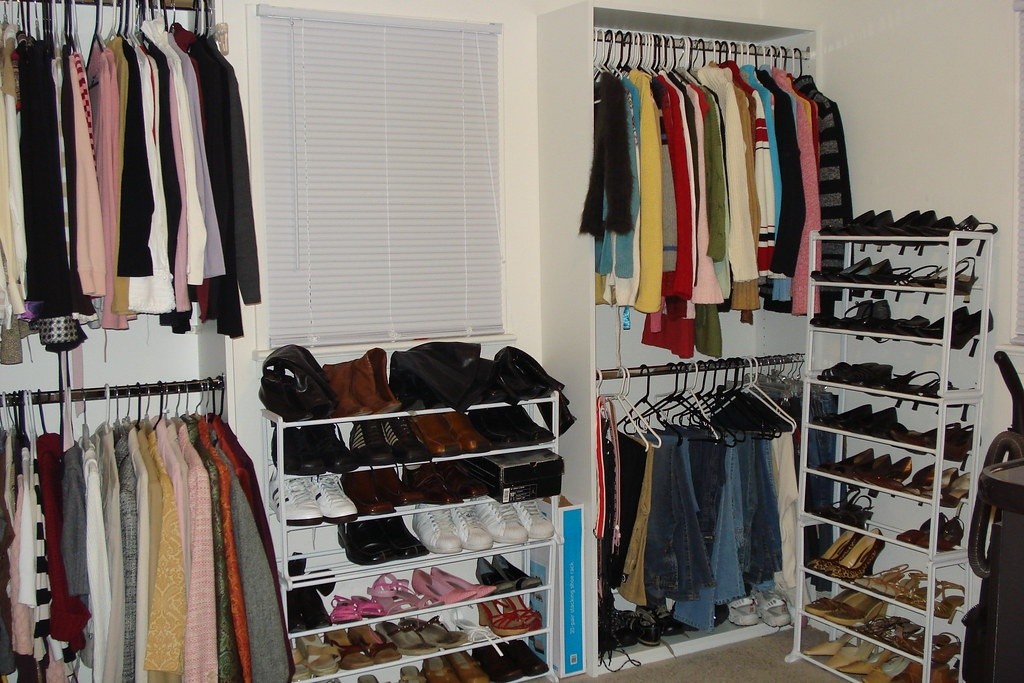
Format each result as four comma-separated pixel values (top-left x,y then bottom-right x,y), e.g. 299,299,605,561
536,0,822,677
260,391,559,683
784,229,993,683
0,0,296,683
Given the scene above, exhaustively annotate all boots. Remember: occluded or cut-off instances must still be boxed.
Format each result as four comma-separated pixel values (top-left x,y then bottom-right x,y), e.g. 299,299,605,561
287,552,336,633
389,342,494,415
322,348,402,419
482,346,577,437
259,345,339,426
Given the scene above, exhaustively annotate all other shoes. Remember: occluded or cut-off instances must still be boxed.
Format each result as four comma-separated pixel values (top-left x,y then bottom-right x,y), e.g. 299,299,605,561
357,639,490,683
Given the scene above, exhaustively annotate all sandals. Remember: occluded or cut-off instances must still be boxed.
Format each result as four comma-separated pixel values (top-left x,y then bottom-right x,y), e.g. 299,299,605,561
367,573,445,616
439,608,509,656
330,595,387,624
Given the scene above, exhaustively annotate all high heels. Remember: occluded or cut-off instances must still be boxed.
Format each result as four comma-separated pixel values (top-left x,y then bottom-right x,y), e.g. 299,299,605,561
804,211,998,682
477,595,542,636
412,567,497,604
476,554,541,594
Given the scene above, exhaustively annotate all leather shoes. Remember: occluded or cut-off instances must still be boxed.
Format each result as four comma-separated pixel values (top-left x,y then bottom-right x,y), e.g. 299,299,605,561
471,405,556,449
340,467,424,516
405,461,488,504
407,411,492,457
472,639,549,683
338,516,429,565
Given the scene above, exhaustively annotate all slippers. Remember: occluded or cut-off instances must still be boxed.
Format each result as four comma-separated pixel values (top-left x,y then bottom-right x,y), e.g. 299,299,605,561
292,617,467,678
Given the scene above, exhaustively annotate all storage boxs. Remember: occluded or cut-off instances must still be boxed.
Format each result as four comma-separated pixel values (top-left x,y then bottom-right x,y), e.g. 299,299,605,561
456,448,564,503
529,495,586,678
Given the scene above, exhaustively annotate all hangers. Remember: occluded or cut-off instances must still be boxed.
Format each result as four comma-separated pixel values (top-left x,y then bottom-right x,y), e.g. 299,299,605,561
0,375,225,487
596,353,825,452
594,27,802,104
0,0,229,56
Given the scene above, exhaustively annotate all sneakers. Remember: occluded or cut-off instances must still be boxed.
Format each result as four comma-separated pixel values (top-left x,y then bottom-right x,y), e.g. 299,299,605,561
412,504,462,554
598,589,794,652
272,424,350,476
448,507,493,552
475,501,529,544
269,474,358,525
349,418,429,466
513,500,555,539
